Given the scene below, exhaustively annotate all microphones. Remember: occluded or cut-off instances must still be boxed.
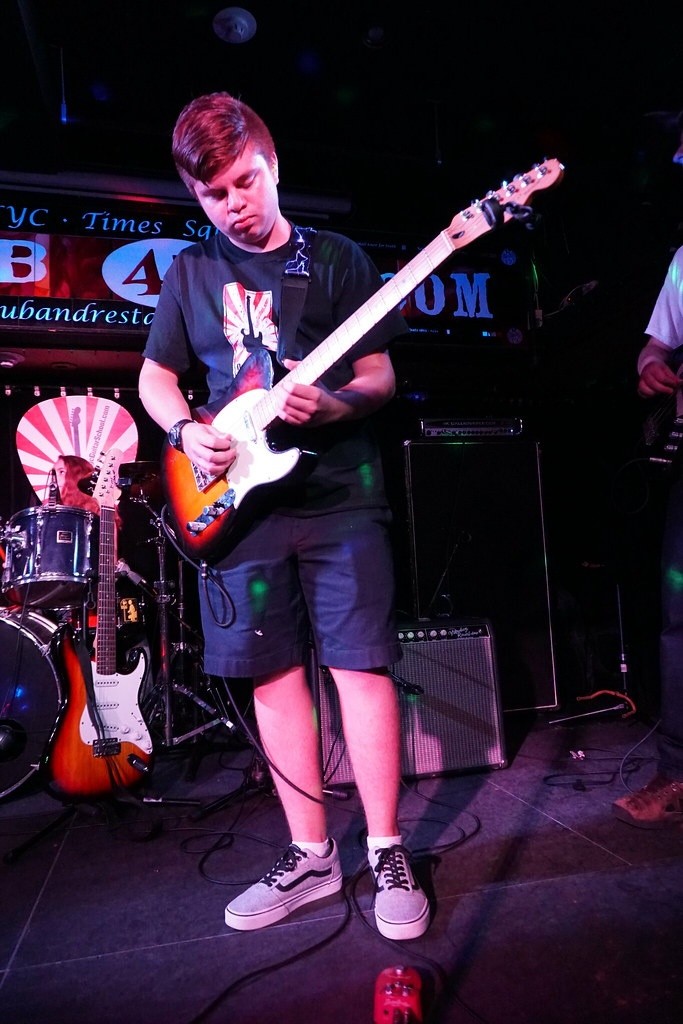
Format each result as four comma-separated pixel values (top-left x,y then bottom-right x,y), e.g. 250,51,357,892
0,722,15,752
51,468,61,502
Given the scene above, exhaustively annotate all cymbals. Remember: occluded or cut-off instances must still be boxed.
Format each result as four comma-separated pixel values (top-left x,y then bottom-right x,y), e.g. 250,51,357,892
117,460,163,499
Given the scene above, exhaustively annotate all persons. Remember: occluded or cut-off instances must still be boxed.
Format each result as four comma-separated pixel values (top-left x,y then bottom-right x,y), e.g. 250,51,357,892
40,455,102,628
608,244,683,829
139,93,433,942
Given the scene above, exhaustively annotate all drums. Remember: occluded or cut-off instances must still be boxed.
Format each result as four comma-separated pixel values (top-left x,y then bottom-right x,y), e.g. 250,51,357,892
0,608,65,799
2,508,99,610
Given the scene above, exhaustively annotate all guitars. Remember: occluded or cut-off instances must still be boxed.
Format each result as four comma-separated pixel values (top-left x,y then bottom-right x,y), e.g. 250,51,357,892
158,155,567,564
634,343,683,480
51,493,157,796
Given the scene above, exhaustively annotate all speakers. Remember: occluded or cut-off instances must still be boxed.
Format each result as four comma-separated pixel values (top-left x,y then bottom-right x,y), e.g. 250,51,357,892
379,438,559,715
308,622,509,788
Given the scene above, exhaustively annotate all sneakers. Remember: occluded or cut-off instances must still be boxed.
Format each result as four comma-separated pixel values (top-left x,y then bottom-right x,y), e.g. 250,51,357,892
224,838,342,931
368,842,431,941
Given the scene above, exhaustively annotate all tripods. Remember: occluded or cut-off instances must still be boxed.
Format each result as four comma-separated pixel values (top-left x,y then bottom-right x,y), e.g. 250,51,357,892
120,480,351,822
547,573,683,747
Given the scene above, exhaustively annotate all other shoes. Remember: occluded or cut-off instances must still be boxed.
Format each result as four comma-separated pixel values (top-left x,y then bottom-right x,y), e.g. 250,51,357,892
613,773,683,830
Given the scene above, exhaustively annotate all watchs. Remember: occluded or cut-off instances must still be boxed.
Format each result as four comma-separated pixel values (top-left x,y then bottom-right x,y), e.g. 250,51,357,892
168,417,194,453
